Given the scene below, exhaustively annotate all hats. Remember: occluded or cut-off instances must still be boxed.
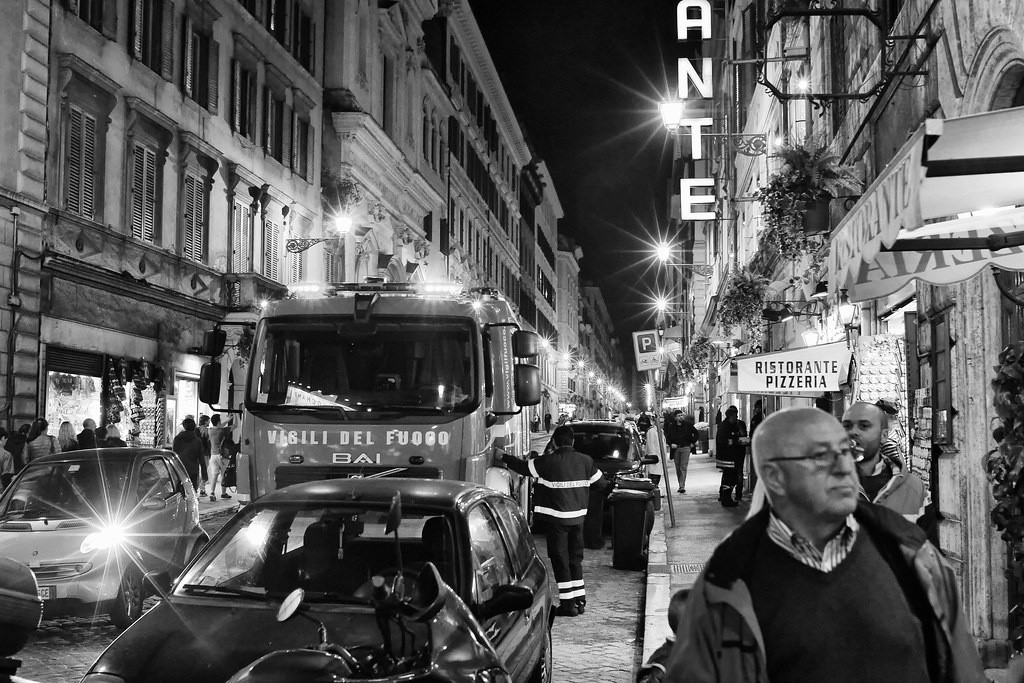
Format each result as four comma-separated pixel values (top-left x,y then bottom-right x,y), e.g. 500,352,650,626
876,397,900,414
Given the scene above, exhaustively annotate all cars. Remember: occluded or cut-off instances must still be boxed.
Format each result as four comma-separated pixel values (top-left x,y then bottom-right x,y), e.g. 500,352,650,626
81,476,557,683
526,412,659,535
0,446,240,631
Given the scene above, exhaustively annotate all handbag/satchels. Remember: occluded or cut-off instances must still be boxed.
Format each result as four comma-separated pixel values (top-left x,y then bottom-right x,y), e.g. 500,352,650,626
222,462,235,487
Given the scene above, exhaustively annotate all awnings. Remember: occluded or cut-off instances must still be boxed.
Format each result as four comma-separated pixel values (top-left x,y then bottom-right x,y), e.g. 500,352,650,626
720,340,856,398
825,106,1024,302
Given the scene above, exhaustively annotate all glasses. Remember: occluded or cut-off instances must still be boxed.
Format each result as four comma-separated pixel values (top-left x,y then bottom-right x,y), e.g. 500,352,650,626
767,448,864,468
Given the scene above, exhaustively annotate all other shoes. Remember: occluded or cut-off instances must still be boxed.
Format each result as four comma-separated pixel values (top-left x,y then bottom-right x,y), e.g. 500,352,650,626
554,606,579,616
221,493,231,498
210,495,216,501
577,605,585,614
677,489,686,493
199,491,207,497
722,500,738,507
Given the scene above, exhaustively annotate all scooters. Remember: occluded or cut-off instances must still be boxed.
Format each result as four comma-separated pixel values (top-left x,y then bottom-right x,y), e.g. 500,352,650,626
221,489,535,682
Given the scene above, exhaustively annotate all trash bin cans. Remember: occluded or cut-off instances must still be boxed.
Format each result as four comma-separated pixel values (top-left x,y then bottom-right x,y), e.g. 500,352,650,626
608,489,654,570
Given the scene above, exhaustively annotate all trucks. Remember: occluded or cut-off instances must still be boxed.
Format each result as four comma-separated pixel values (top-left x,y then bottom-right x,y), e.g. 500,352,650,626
198,275,551,528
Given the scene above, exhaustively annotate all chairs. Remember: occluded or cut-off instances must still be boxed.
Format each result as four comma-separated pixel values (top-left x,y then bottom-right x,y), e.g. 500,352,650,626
303,512,357,569
121,462,164,504
421,518,457,595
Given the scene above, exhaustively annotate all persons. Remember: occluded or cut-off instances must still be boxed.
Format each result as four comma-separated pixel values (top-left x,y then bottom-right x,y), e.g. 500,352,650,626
638,418,668,485
843,401,944,547
637,589,694,683
748,399,763,493
636,412,651,437
0,417,128,512
534,413,540,433
714,405,747,507
665,411,700,493
171,412,238,502
545,410,552,434
877,396,911,469
663,408,677,432
493,427,605,616
698,406,704,421
662,406,989,683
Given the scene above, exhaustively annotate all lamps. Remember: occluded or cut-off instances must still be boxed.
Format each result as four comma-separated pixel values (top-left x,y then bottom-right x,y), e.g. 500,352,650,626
286,210,354,253
781,278,862,351
654,243,714,277
657,98,766,156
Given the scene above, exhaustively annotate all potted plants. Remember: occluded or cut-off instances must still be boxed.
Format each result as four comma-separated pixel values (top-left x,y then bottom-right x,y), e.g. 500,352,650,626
753,137,866,265
